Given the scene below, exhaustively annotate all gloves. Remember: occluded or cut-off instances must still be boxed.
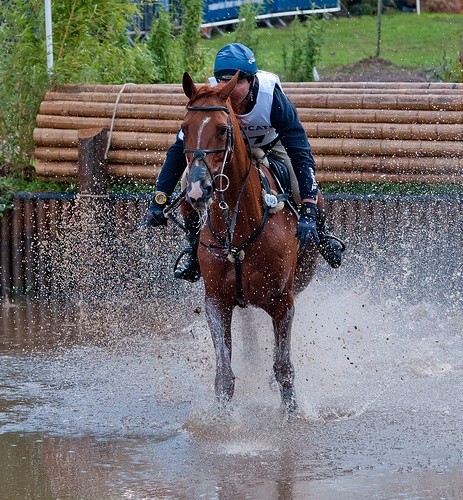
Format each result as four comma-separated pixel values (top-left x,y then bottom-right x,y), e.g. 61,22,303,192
297,203,320,246
148,194,172,227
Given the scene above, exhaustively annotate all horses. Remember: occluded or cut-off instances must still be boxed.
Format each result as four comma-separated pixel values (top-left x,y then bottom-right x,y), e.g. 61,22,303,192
180,71,324,422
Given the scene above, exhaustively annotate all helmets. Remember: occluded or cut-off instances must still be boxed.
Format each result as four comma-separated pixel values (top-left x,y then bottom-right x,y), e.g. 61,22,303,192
214,43,258,76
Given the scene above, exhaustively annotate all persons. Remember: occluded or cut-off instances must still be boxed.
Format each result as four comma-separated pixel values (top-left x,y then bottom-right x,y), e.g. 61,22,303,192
145,43,345,279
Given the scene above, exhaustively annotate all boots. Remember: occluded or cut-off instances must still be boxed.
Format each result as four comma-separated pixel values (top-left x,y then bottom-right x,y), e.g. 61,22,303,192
317,208,341,268
174,214,201,281
